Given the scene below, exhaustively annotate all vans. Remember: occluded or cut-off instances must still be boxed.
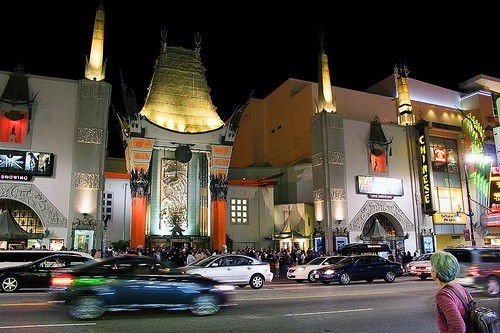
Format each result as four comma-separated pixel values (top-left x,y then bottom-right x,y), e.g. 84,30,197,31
443,245,500,297
333,243,395,262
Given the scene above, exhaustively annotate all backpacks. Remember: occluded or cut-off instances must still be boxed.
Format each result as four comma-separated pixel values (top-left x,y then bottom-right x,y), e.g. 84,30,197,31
437,285,500,333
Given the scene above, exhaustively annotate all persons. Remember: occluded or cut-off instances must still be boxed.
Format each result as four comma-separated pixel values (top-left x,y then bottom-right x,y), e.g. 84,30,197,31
0,244,420,276
430,251,478,333
27,152,49,172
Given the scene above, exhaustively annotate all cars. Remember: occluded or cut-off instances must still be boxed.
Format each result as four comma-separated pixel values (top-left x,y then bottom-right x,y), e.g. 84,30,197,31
287,256,347,283
47,255,240,321
0,253,95,293
313,255,404,287
405,253,433,280
176,254,274,289
